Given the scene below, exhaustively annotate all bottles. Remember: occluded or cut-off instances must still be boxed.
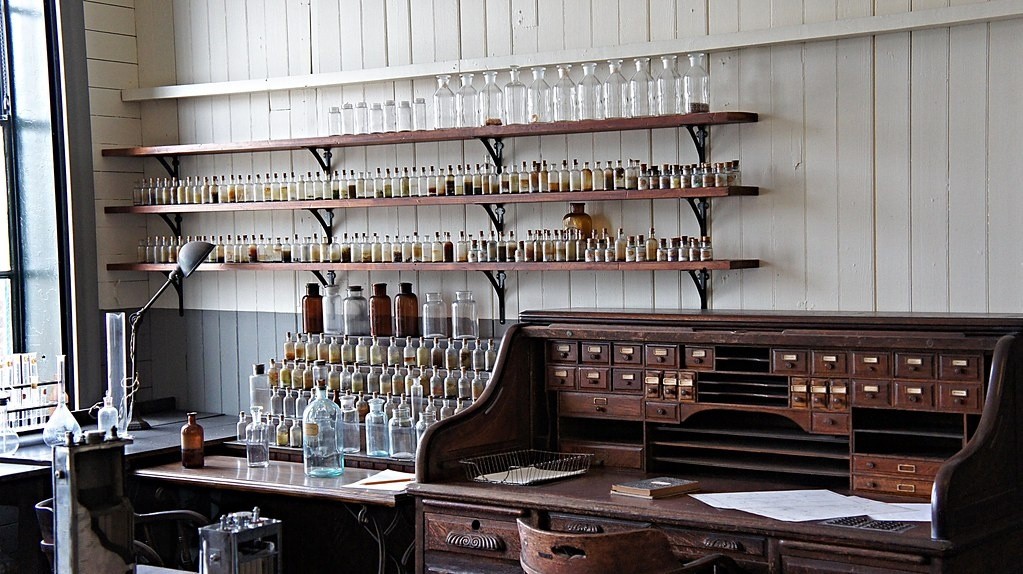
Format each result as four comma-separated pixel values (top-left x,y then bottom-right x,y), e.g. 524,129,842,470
180,412,205,469
42,354,82,448
246,406,270,468
134,154,742,262
433,53,711,129
97,390,118,440
328,98,425,136
0,390,20,457
238,283,496,459
303,387,344,479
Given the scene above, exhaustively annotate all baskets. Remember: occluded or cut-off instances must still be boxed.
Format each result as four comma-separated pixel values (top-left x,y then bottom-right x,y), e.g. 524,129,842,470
459,449,594,487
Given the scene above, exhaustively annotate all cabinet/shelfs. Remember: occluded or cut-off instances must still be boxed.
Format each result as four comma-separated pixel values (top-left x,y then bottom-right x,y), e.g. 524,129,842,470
406,312,1022,574
100,103,760,310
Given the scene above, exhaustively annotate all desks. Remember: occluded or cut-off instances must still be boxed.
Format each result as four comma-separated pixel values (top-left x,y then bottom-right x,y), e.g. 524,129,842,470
133,454,415,574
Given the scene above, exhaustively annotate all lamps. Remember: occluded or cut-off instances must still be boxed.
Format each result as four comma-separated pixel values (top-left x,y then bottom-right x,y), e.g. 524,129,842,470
121,239,216,431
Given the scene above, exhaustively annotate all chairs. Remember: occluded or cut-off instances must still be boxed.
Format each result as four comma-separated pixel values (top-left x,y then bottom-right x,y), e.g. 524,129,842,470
34,496,209,573
516,516,736,574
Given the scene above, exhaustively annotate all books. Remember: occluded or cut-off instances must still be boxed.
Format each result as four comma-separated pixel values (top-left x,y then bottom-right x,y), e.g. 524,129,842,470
610,477,700,499
